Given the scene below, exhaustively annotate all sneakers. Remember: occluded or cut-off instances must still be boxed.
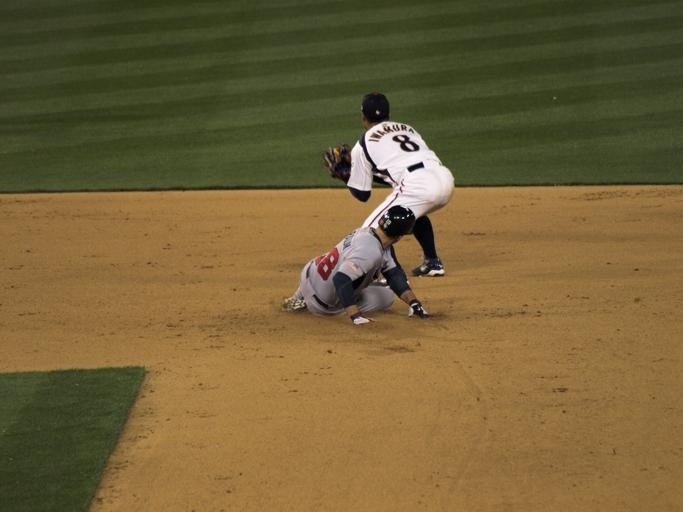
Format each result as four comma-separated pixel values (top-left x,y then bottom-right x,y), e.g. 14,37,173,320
286,299,307,313
411,257,445,277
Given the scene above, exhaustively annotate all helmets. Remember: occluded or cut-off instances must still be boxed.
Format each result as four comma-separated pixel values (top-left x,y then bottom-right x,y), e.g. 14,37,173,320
379,205,415,236
361,91,390,114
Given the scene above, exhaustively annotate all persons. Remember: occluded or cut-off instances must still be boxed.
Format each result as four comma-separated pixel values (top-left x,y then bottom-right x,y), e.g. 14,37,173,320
278,205,427,327
324,92,453,277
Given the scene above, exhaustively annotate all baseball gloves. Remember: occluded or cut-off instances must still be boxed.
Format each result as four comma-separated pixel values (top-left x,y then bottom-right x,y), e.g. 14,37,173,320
321,145,350,178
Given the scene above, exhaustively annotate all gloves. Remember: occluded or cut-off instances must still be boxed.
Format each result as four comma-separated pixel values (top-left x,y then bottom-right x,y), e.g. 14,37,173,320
408,300,431,319
351,313,376,325
